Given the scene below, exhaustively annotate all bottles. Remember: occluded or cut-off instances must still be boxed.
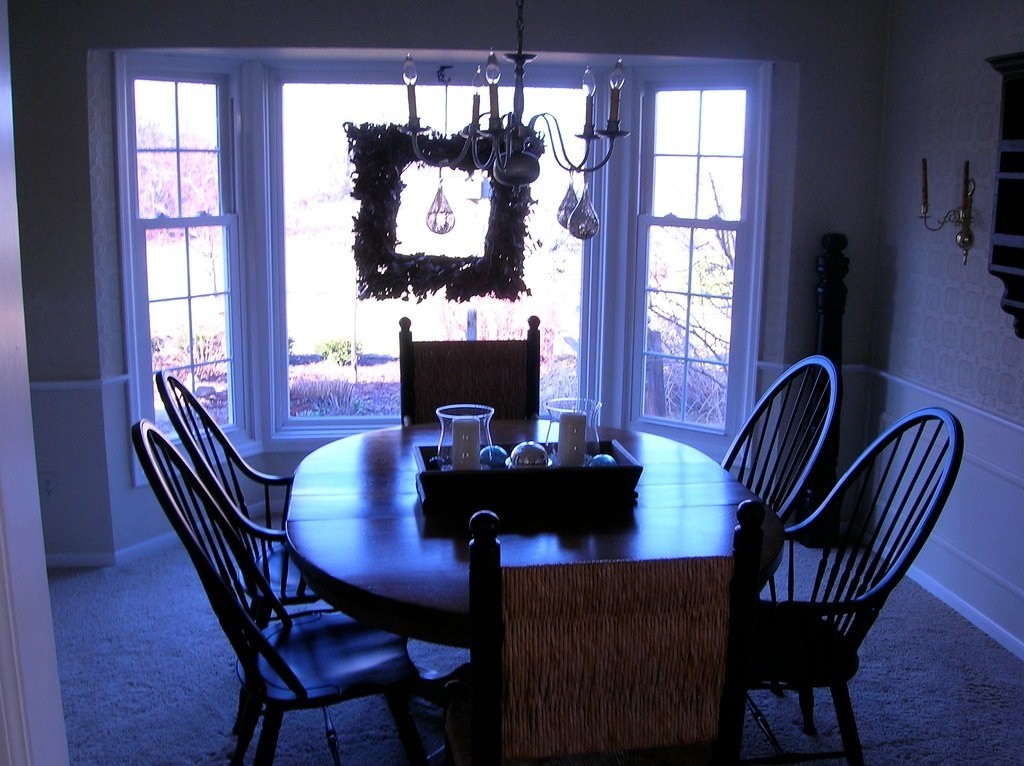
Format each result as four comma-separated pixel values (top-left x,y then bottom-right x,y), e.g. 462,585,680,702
436,405,495,471
546,398,603,467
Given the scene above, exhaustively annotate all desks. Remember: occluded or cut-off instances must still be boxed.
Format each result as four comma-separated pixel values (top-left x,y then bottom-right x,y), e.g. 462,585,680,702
285,418,785,724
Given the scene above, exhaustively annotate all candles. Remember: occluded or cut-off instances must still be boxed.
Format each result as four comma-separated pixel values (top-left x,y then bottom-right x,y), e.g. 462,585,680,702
557,411,586,466
922,157,929,206
962,160,969,210
451,418,481,471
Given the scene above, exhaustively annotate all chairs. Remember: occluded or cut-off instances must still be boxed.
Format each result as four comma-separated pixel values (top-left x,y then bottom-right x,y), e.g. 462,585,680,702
740,406,964,766
444,499,765,766
399,316,541,425
721,355,842,694
131,369,428,766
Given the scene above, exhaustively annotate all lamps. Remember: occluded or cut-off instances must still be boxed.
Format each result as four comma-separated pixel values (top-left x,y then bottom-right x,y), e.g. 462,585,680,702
396,0,633,239
